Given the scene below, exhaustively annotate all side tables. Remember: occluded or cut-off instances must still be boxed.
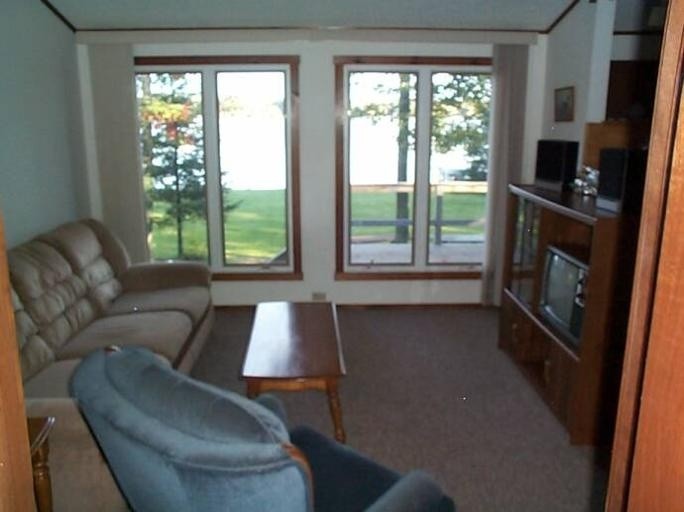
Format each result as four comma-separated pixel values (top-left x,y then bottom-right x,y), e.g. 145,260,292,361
27,416,58,512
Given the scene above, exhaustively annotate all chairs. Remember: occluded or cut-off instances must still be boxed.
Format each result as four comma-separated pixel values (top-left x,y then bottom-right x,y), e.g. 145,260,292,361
69,346,455,512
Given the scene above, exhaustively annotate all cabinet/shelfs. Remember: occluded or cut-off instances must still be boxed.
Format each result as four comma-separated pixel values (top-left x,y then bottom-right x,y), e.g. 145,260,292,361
498,183,623,475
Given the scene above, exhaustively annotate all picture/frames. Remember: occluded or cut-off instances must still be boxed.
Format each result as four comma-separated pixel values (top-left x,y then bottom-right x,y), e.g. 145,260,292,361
554,86,575,121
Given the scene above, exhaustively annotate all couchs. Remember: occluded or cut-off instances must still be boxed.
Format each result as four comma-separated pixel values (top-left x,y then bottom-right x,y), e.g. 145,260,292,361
8,217,217,398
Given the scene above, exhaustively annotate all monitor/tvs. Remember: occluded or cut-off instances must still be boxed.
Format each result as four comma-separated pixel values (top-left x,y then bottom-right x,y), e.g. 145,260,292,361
537,245,589,348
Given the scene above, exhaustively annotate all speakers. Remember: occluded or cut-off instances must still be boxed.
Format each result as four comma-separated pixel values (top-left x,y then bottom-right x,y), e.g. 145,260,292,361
535,140,578,193
596,148,647,217
